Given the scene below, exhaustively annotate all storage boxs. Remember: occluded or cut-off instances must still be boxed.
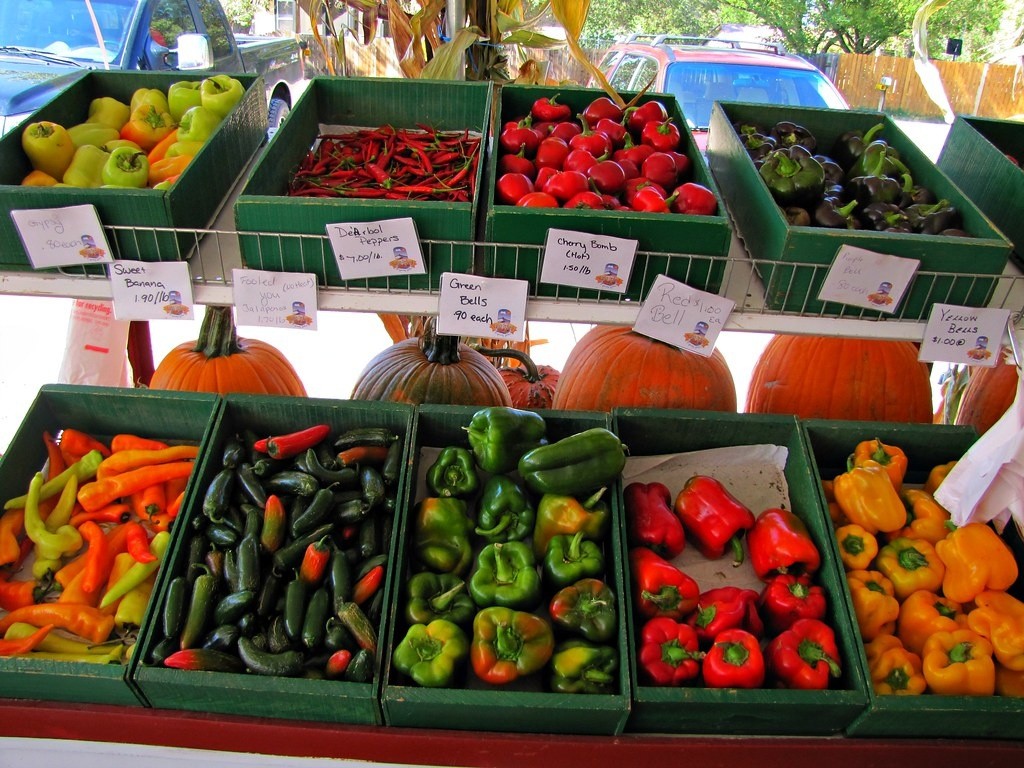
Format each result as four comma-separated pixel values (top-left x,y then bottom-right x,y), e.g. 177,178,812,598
0,68,1022,742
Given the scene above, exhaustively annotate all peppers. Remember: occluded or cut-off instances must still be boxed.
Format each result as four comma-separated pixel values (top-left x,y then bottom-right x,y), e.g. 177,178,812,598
0,407,1024,692
23,73,967,236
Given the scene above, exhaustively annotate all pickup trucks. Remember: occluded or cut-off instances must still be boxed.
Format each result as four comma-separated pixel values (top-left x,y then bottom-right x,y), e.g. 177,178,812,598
0,0,306,144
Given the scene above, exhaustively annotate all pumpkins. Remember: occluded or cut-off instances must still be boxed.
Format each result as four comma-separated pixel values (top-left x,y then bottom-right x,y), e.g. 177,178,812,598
150,307,1017,437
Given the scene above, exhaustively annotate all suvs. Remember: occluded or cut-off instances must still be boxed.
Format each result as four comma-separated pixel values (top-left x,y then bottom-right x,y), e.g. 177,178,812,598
585,30,852,152
